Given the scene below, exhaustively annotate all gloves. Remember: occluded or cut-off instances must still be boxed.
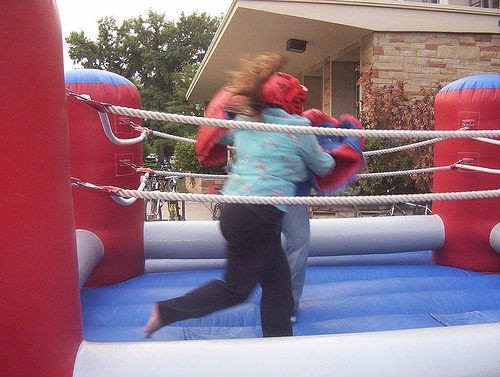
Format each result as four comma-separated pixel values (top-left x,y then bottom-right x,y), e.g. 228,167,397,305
192,83,256,167
299,107,367,195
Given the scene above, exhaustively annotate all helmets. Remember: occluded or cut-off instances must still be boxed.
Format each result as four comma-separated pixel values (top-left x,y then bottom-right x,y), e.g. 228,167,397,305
258,70,309,117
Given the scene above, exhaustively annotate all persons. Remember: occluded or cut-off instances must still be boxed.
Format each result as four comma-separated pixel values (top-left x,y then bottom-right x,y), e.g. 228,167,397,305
279,174,319,322
143,52,339,339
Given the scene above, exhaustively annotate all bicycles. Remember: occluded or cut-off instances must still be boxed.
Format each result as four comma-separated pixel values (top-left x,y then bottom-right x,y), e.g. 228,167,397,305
143,173,163,222
160,176,186,222
371,184,432,217
